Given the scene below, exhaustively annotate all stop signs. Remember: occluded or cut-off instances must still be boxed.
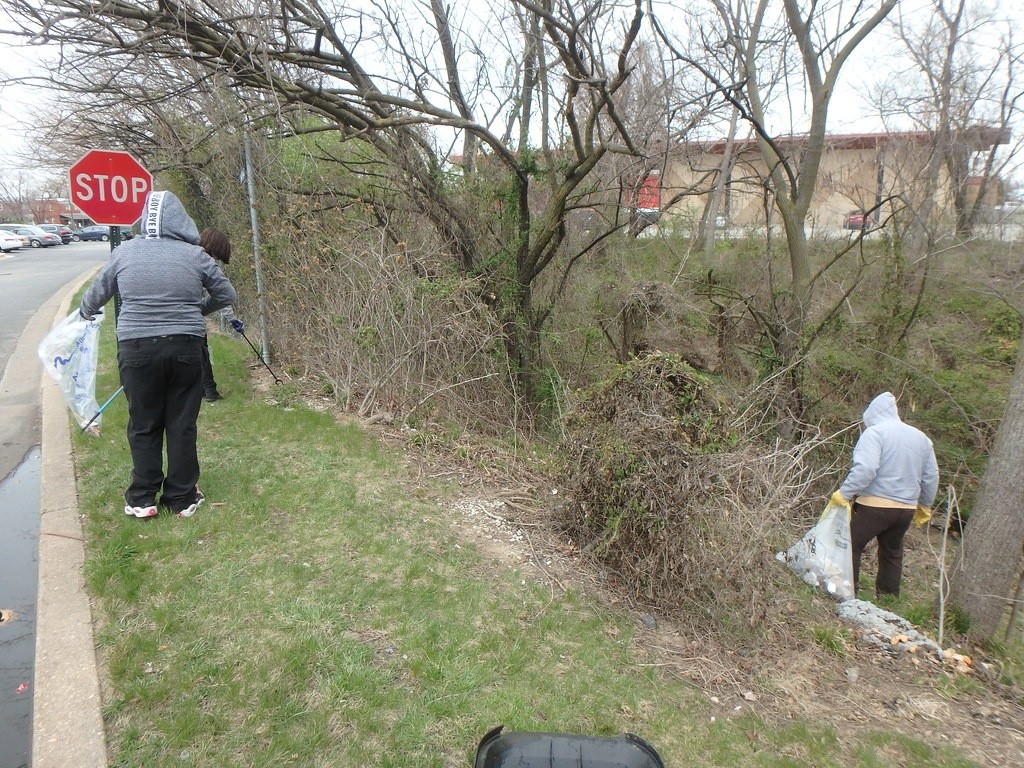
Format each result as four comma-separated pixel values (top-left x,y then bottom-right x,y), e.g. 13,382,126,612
67,149,152,226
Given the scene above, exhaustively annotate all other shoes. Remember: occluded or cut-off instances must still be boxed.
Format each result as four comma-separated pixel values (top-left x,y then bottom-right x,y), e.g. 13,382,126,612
206,393,223,402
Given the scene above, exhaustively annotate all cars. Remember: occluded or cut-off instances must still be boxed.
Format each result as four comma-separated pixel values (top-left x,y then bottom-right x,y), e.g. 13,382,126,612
7,231,31,246
9,228,62,248
117,228,134,241
72,226,112,241
0,233,22,253
713,210,729,230
843,208,871,231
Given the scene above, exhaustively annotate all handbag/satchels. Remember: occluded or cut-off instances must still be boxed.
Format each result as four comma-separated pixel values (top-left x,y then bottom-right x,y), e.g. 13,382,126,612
39,302,105,437
775,506,850,600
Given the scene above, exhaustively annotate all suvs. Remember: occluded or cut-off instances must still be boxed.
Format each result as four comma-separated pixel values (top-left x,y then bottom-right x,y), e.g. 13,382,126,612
37,223,72,244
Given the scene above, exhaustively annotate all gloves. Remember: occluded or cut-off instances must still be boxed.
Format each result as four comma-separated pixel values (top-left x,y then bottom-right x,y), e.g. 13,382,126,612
79,298,104,320
229,319,246,334
912,505,932,526
830,489,849,507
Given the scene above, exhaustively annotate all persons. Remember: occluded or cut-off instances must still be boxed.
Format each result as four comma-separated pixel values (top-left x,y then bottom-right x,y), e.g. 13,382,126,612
79,191,236,519
829,391,939,598
199,227,236,402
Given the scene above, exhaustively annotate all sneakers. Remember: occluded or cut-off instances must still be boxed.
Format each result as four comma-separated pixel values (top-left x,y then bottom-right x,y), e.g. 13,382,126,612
124,501,158,518
160,490,206,518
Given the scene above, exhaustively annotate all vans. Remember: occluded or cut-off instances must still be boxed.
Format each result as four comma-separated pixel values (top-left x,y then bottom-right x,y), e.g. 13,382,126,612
0,224,43,235
